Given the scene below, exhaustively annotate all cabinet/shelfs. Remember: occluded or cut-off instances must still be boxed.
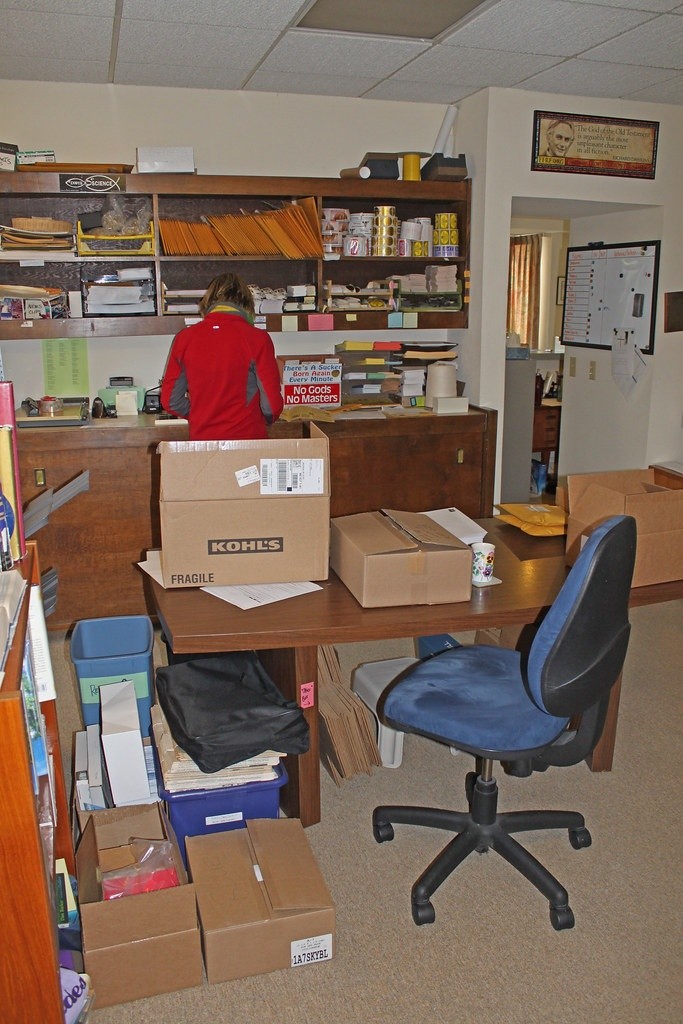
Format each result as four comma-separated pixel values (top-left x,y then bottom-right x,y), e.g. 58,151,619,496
532,398,562,485
339,352,404,406
0,171,472,340
0,540,76,1024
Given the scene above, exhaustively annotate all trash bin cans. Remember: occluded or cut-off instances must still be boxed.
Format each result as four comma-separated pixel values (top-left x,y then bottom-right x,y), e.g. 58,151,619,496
68,614,156,741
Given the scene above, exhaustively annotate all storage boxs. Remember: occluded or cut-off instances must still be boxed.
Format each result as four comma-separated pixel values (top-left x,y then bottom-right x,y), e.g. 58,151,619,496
330,509,473,608
75,802,204,1010
421,153,468,181
148,724,288,870
159,420,331,588
72,736,169,846
185,818,335,983
419,634,463,662
276,355,342,408
565,468,683,588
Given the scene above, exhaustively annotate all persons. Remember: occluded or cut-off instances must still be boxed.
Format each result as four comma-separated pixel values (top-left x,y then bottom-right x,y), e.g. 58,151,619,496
160,271,284,442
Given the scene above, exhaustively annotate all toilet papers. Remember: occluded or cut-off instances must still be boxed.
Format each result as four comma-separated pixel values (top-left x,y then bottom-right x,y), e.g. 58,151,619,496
426,363,457,408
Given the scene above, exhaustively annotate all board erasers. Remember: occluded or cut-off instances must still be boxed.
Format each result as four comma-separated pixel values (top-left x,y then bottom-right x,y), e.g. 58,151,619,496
632,294,645,317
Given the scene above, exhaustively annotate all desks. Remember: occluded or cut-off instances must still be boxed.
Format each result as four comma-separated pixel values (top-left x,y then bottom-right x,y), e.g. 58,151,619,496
150,519,683,828
532,352,565,374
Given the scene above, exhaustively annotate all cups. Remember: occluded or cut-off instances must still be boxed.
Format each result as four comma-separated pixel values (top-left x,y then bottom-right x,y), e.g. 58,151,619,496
471,543,495,582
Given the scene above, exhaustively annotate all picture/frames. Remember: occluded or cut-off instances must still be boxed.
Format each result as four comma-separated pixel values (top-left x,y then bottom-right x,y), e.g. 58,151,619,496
556,276,566,304
531,110,660,180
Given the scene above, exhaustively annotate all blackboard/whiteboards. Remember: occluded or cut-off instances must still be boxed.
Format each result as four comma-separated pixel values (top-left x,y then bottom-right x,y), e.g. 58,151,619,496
560,239,662,357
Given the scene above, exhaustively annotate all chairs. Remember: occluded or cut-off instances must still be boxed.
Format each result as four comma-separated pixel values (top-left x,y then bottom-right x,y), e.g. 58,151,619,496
373,516,638,932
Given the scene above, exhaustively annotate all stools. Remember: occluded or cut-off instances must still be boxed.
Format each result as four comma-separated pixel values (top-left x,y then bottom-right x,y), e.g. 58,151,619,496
351,657,460,769
